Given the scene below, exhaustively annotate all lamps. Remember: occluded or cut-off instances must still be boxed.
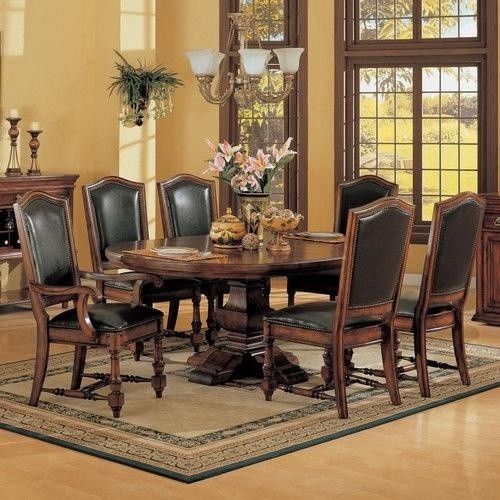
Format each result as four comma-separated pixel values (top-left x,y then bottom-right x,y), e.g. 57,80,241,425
185,0,305,108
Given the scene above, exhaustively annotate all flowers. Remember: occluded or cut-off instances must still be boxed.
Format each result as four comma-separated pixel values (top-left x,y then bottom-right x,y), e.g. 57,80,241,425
203,137,297,195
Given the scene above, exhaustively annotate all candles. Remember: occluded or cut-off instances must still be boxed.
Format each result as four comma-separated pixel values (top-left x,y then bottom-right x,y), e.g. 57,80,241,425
9,109,19,117
31,120,40,130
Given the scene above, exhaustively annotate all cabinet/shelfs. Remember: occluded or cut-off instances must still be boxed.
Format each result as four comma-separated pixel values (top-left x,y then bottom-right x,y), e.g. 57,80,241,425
471,192,500,325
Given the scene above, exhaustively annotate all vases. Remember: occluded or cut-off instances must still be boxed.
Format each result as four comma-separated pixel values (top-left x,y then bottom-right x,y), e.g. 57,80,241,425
238,192,269,235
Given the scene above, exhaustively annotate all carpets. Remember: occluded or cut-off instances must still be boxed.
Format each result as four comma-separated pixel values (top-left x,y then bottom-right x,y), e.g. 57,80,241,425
0,329,500,484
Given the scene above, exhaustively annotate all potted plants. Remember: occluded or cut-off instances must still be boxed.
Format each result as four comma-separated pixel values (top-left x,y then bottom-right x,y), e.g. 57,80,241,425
103,47,184,128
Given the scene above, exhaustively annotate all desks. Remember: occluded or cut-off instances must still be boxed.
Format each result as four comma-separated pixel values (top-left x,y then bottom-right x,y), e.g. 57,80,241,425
0,175,81,310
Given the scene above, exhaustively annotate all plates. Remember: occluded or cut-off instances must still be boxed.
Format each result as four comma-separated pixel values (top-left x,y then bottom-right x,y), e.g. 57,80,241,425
149,246,198,258
301,231,344,240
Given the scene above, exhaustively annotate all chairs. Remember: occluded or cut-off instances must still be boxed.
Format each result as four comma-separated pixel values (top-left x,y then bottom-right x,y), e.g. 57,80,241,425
12,188,167,419
261,196,416,421
156,174,230,338
394,189,487,396
81,175,204,362
286,174,398,305
